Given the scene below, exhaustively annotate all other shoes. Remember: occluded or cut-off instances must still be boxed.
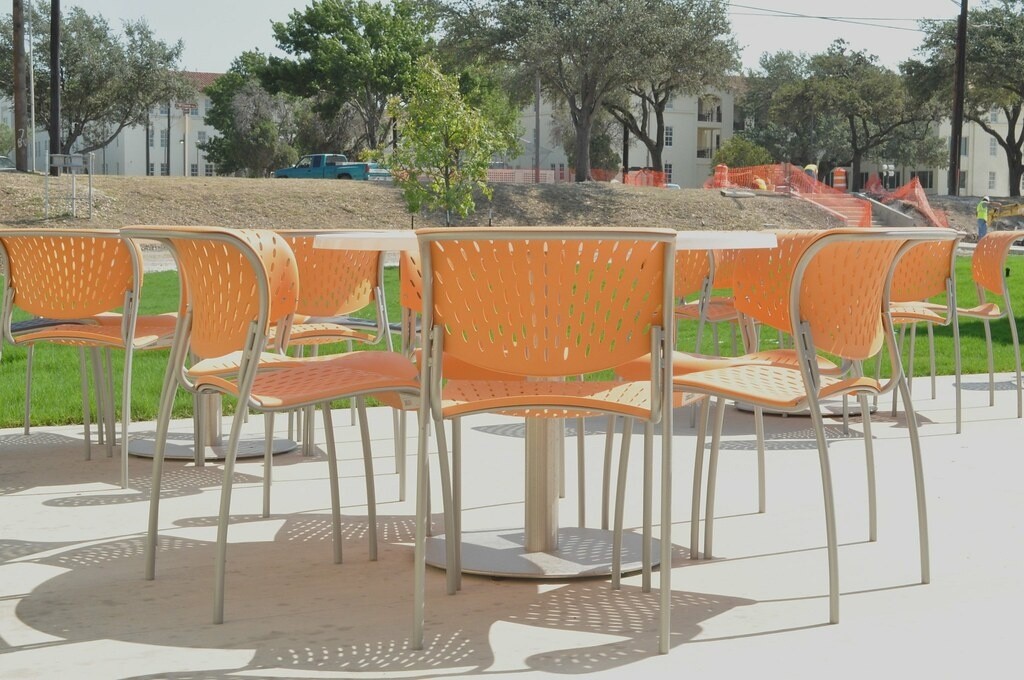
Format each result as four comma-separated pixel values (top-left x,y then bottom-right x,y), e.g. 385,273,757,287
812,192,816,194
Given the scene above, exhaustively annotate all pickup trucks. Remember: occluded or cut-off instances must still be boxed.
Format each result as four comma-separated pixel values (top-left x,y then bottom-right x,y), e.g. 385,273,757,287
271,154,370,180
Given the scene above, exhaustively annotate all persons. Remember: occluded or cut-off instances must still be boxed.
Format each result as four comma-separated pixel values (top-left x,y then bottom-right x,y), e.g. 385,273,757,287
976,195,1003,239
751,175,768,190
803,163,819,182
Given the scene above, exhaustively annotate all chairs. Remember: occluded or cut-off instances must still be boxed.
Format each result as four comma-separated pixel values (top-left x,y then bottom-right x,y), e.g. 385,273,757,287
0,224,1023,655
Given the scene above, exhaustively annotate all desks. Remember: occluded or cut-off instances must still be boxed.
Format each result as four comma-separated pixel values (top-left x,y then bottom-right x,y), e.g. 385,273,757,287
312,230,777,578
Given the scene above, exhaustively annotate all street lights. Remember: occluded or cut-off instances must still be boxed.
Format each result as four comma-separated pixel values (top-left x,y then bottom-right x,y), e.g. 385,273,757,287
883,163,895,191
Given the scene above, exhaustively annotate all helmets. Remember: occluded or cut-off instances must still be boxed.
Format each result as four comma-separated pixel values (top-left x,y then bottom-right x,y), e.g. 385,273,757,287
753,175,760,179
982,195,989,201
